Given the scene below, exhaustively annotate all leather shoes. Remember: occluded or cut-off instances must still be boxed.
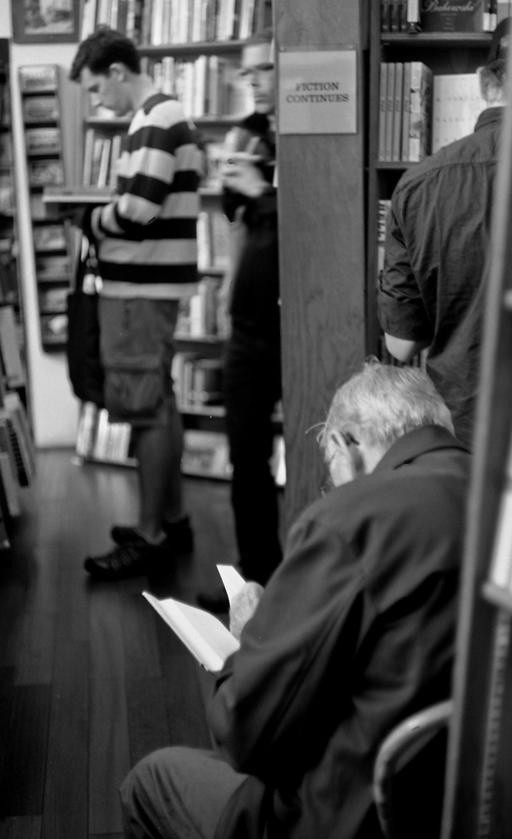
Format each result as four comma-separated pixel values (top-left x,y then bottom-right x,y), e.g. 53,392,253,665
83,512,194,579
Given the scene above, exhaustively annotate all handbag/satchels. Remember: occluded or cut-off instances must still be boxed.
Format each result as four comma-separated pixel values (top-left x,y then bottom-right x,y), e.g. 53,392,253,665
66,291,105,407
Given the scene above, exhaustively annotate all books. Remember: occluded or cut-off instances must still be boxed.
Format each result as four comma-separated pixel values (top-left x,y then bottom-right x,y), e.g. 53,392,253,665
1,0,275,408
378,60,492,161
375,0,512,34
377,200,392,241
139,563,257,677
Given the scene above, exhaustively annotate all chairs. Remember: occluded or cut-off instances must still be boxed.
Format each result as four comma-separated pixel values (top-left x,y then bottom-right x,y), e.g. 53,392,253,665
373,700,452,838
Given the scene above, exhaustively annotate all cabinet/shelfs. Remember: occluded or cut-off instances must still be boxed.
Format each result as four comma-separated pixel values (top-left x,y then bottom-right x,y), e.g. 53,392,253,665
71,2,287,487
367,0,499,368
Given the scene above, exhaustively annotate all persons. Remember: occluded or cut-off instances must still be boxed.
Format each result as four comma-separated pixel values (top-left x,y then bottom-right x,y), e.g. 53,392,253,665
196,26,286,613
119,357,511,837
60,26,206,585
